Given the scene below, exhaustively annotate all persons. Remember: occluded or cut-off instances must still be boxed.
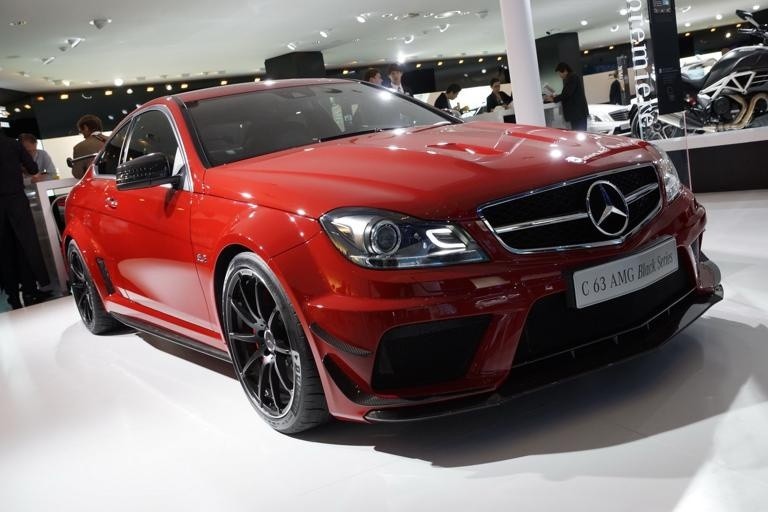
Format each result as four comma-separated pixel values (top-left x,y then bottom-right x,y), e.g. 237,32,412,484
72,114,109,179
0,134,53,309
544,62,589,131
609,73,622,105
487,78,513,112
18,133,64,234
385,67,413,96
365,70,383,85
435,84,461,116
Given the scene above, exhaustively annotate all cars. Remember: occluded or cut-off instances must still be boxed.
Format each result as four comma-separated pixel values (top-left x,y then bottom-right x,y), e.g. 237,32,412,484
62,76,723,437
585,103,631,136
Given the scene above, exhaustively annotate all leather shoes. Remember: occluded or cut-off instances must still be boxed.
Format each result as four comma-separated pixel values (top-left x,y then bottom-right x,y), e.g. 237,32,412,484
7,288,51,304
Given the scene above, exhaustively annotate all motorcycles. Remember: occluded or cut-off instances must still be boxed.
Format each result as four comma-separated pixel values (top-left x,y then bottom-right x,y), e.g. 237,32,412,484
630,10,768,139
